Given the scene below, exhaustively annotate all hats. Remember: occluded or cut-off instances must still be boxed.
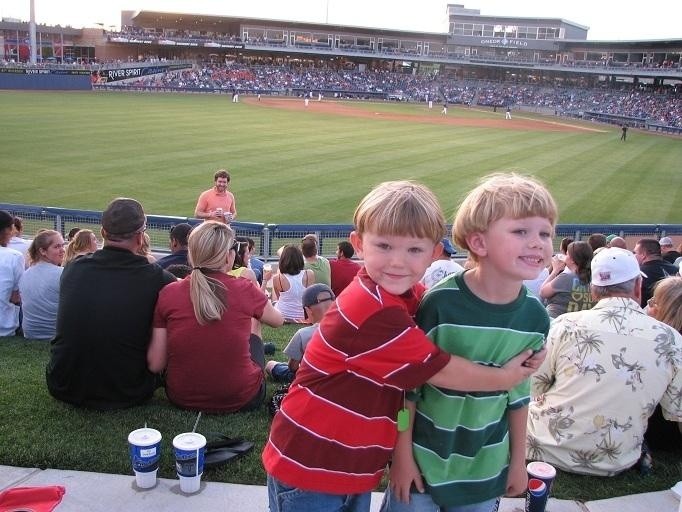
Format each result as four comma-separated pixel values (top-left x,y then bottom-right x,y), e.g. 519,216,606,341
440,238,456,254
658,237,673,245
102,198,145,234
590,247,649,287
302,283,335,320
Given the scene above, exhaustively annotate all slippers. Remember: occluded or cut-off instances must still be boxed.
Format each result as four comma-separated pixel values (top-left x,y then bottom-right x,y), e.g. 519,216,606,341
204,432,255,467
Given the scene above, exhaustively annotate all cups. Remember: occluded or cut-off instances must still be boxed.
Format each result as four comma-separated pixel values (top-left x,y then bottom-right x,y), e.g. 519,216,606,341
171,431,207,495
556,253,565,270
127,427,162,490
263,264,272,270
224,212,232,224
525,461,556,512
216,208,222,218
